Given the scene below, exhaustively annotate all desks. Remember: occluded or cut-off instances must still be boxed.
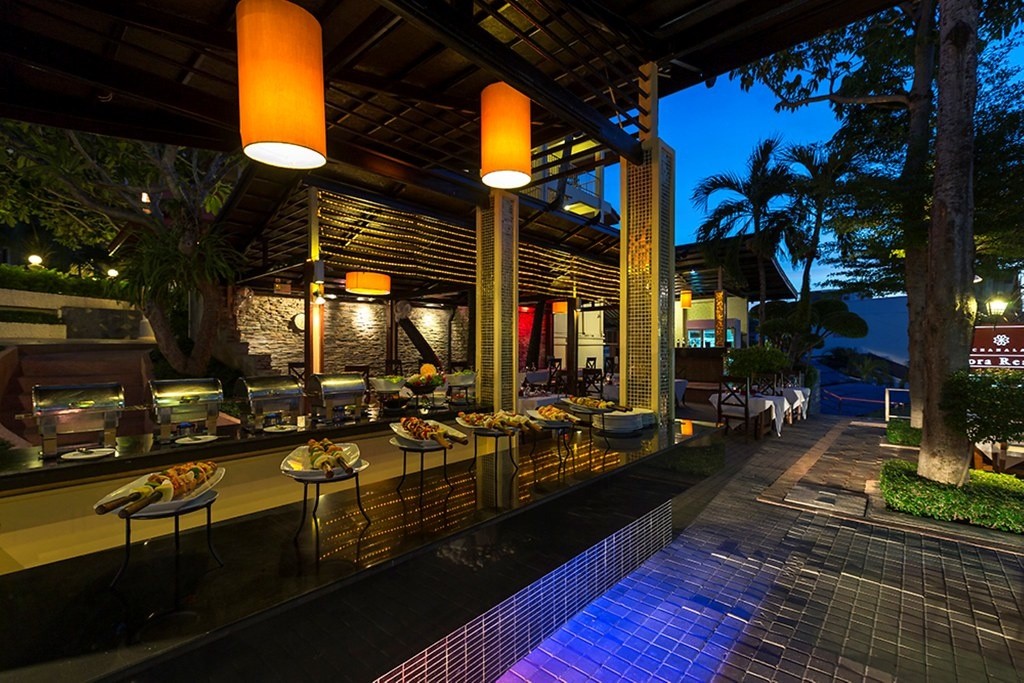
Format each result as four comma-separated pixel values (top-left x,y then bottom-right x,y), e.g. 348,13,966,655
675,378,688,408
602,382,619,400
750,384,805,424
519,389,571,414
552,367,583,377
708,393,790,441
522,367,549,385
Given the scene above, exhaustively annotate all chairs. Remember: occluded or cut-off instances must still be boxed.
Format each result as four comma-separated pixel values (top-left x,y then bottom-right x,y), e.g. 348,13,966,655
718,369,802,440
417,357,437,373
521,357,605,397
448,361,470,373
287,359,304,377
345,364,370,392
385,359,404,376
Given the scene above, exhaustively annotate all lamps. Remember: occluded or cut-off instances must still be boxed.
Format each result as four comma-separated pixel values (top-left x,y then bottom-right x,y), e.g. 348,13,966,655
552,302,568,314
313,260,326,304
345,271,391,296
680,290,692,310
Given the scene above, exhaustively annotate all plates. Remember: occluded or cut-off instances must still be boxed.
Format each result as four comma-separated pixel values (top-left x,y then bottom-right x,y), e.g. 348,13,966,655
526,409,581,422
175,435,218,444
390,419,467,445
455,417,482,428
560,397,586,407
592,408,656,433
93,466,226,516
279,442,361,476
263,425,297,432
61,448,115,460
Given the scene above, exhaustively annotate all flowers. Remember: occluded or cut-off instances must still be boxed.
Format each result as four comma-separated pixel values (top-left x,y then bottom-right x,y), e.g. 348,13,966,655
481,82,531,189
236,0,328,170
